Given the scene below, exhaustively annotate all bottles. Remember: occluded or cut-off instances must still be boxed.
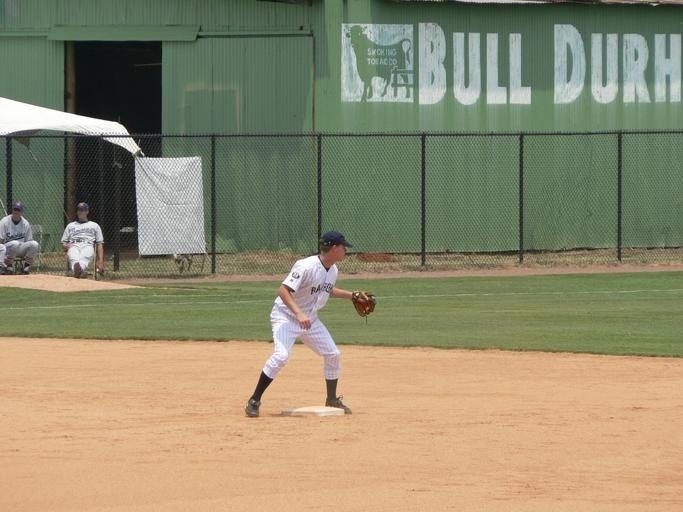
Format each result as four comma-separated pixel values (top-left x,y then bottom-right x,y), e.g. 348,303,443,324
319,231,354,247
13,203,22,211
77,203,88,210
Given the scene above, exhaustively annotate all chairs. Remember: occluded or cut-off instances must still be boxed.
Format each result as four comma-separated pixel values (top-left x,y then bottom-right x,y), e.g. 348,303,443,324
74,262,82,277
326,397,352,414
245,397,260,417
22,270,30,275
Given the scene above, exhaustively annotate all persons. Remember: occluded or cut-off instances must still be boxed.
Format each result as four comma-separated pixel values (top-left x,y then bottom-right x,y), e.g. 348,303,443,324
244,230,377,418
0,202,39,274
60,202,104,279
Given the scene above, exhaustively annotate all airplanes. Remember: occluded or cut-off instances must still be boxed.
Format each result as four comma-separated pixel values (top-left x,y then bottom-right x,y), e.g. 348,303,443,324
352,291,376,324
